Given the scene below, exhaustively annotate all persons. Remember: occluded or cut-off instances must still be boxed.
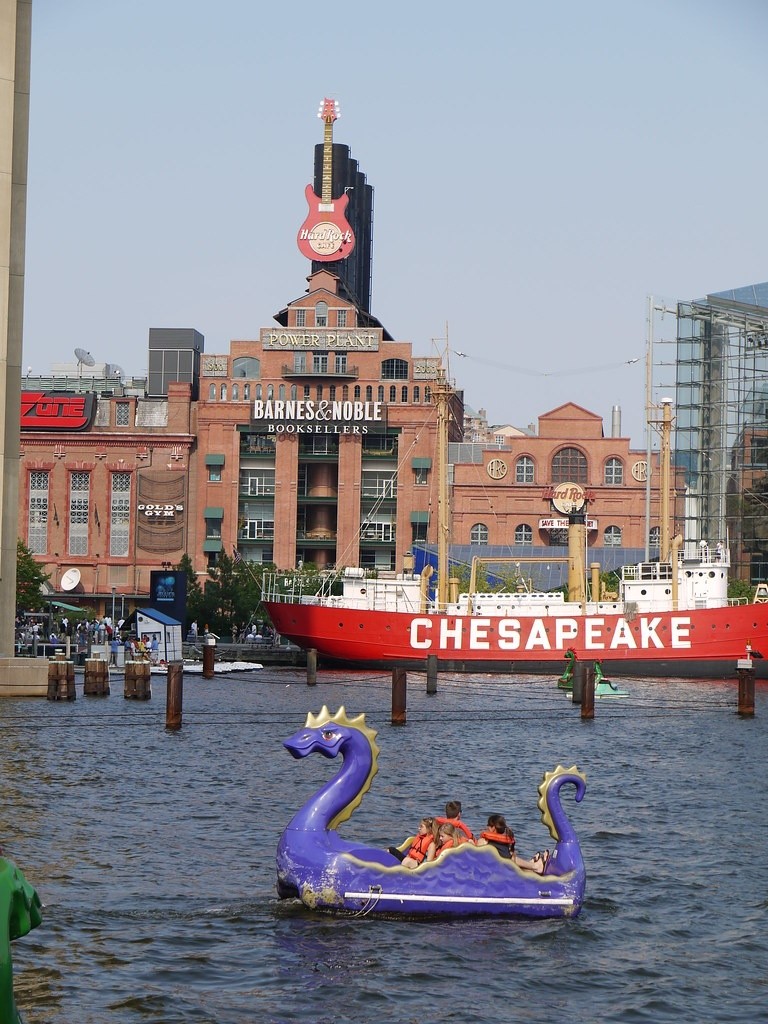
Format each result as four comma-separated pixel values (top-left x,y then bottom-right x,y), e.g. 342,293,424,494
15,609,111,653
701,541,709,563
109,637,121,667
435,823,474,859
232,621,273,643
204,622,208,634
191,620,197,635
118,618,125,628
104,623,112,641
436,802,549,874
477,815,515,860
387,817,436,869
120,635,158,660
715,541,723,563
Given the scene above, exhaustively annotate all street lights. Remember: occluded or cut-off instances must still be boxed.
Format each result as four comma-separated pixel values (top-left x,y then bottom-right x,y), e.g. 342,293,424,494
120,592,126,617
110,585,118,641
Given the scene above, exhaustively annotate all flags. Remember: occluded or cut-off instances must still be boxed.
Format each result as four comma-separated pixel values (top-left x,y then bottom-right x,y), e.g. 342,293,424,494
232,549,241,564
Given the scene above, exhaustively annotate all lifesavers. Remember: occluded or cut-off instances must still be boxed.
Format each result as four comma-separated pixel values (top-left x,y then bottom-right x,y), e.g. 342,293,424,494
481,831,517,845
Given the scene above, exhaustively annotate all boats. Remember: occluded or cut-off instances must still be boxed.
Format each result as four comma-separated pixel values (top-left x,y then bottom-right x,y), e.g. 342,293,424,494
557,647,630,699
262,535,768,679
276,704,587,918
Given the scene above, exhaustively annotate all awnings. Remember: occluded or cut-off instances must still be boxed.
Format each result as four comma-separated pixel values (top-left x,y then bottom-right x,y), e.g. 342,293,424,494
204,507,223,518
411,511,429,524
204,540,222,552
411,457,431,469
206,454,224,466
46,601,85,612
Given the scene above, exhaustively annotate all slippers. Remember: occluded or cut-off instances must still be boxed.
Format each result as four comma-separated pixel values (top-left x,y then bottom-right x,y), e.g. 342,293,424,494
532,849,550,875
